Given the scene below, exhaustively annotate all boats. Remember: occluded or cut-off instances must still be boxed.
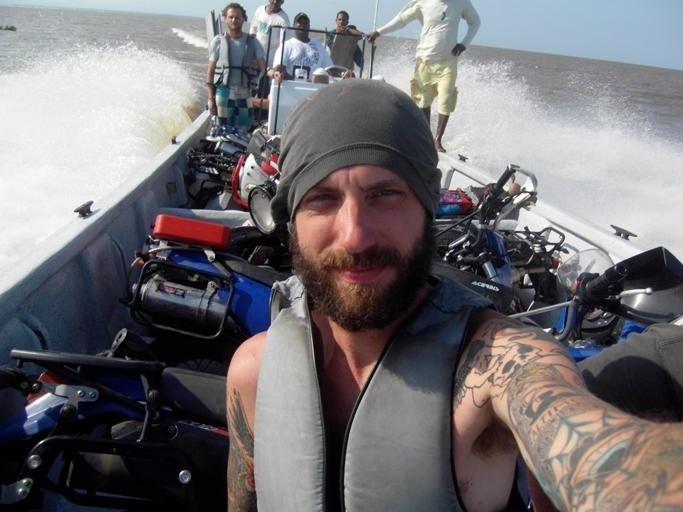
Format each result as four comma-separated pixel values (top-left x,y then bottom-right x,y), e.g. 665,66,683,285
0,23,683,511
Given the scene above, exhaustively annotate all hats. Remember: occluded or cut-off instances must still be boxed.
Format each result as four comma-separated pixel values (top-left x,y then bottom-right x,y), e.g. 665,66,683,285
269,78,443,225
294,12,310,22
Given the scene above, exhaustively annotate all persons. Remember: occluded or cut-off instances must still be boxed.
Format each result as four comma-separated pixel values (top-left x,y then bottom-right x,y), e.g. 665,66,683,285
366,0,480,152
226,79,683,512
326,11,363,71
205,0,290,127
273,12,334,80
